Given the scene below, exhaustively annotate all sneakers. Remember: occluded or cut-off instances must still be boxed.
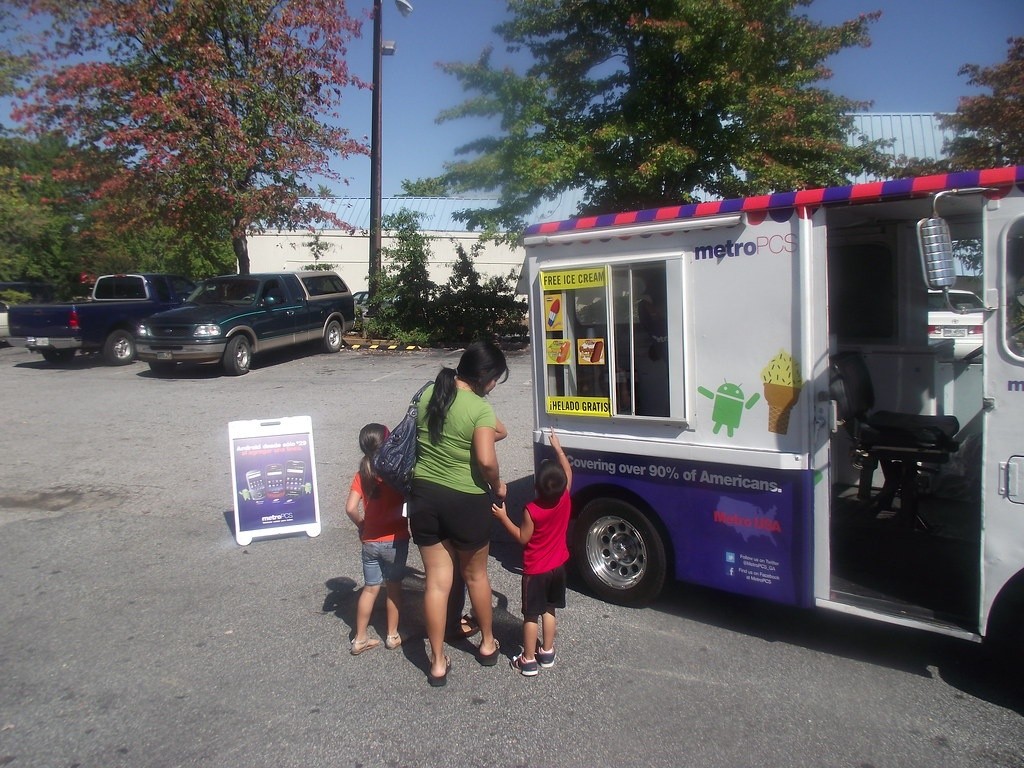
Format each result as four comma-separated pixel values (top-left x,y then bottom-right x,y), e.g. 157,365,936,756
536,645,556,668
509,645,538,675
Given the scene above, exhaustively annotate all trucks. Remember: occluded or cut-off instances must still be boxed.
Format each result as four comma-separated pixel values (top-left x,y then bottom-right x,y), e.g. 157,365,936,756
520,163,1023,712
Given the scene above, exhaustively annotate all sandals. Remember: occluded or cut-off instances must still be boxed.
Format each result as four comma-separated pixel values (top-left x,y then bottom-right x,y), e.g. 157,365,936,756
350,636,383,654
445,613,479,642
385,632,408,649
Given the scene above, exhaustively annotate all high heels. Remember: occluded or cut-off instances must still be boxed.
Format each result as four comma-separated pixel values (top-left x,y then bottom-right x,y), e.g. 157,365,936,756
479,638,500,665
428,655,451,687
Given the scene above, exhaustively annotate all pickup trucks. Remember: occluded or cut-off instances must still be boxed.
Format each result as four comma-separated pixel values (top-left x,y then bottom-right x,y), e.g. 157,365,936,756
133,269,356,378
7,273,196,366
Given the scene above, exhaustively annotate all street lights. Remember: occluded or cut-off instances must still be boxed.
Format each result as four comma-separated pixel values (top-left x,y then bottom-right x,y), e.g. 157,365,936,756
368,0,414,301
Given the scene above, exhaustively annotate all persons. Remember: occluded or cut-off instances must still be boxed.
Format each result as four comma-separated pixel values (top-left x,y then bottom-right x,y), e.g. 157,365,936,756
492,426,573,676
346,423,410,655
411,343,508,686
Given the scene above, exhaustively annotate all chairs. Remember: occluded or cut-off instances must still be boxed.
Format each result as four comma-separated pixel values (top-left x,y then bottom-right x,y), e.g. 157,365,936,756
830,349,961,531
261,285,288,306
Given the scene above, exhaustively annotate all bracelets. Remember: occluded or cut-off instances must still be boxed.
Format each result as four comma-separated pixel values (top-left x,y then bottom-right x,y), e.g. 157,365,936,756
557,453,564,457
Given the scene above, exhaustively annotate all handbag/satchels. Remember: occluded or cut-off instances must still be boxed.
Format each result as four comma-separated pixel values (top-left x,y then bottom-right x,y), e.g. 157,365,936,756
370,381,435,500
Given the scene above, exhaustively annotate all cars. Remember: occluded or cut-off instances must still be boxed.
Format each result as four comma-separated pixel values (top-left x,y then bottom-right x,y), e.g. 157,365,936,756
352,290,370,306
0,300,16,339
